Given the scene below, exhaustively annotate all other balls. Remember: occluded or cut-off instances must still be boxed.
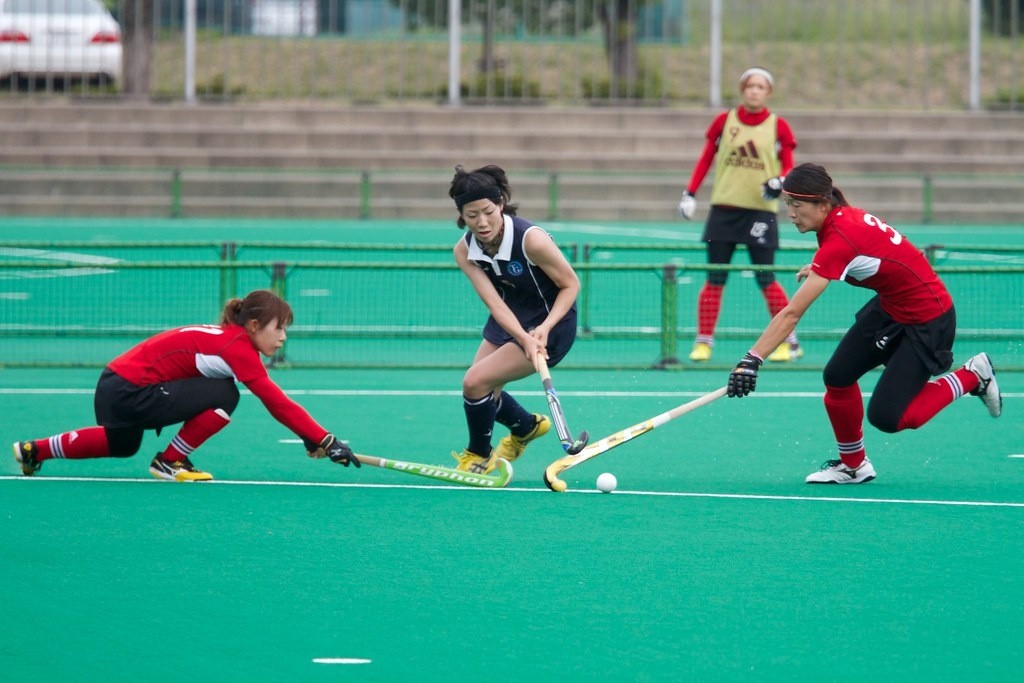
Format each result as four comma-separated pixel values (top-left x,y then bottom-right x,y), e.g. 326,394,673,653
595,472,618,493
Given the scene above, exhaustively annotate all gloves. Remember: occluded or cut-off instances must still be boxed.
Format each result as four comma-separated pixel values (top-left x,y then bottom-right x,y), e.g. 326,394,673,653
759,176,782,200
727,352,763,398
679,193,696,220
305,433,362,470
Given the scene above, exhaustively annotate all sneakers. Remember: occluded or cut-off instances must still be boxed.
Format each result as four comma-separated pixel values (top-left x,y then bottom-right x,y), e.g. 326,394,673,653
496,413,550,462
768,342,803,361
806,456,876,484
452,447,498,476
149,451,214,481
13,441,43,476
689,341,710,362
963,352,1002,418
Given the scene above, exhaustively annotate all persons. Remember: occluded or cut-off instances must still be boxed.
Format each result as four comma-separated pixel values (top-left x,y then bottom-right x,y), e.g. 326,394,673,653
679,68,803,363
13,290,361,482
727,163,1003,485
449,164,579,479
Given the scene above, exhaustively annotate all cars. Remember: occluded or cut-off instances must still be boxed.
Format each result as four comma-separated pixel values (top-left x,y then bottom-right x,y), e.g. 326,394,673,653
0,0,124,86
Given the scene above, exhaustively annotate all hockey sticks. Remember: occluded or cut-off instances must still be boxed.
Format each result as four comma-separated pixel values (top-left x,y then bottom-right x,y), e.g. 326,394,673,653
316,450,514,487
543,386,733,491
527,327,588,455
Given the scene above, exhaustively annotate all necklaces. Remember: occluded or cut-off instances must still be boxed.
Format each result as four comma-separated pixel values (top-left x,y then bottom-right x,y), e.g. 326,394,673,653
478,222,504,251
